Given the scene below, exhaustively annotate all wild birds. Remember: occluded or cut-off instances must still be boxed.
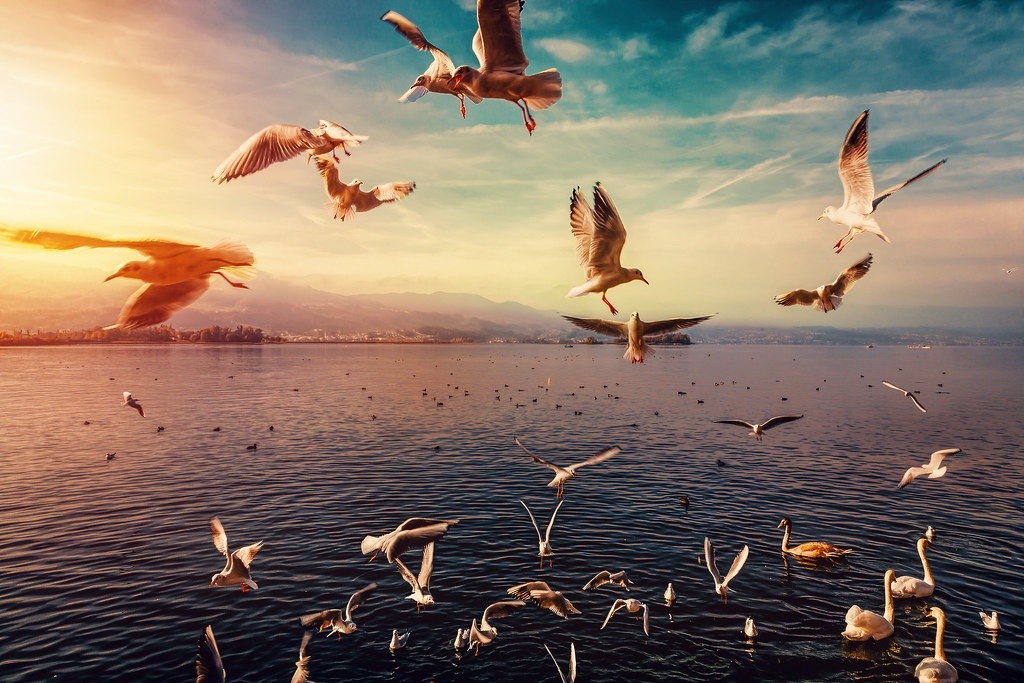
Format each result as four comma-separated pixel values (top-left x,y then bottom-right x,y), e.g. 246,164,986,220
208,119,416,221
380,0,563,136
3,227,255,333
817,107,947,254
563,181,650,316
561,312,719,365
45,348,1002,683
774,256,872,311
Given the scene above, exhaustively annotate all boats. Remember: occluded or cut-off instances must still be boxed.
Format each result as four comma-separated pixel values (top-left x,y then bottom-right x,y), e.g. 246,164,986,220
564,342,573,348
907,344,932,349
865,344,874,349
